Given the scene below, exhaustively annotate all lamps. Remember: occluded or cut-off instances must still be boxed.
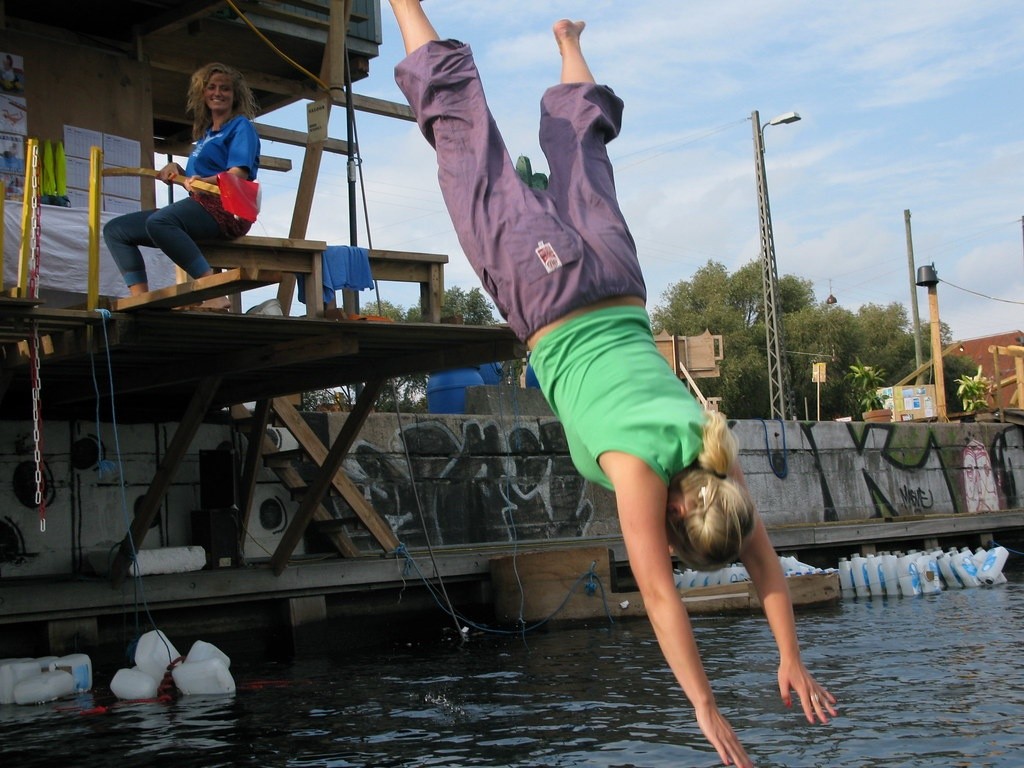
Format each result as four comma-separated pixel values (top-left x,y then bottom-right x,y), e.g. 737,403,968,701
826,280,837,304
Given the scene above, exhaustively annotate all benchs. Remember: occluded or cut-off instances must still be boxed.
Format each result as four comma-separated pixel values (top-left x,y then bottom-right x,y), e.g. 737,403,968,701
178,232,448,323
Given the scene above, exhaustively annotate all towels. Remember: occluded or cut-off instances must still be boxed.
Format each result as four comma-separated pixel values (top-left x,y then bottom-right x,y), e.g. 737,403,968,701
296,245,374,303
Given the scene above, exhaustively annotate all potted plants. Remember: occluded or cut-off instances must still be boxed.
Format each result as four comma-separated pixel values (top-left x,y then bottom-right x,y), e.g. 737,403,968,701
841,357,891,423
953,364,997,423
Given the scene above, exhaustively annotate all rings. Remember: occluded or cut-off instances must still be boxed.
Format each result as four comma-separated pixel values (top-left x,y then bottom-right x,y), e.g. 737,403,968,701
811,694,819,701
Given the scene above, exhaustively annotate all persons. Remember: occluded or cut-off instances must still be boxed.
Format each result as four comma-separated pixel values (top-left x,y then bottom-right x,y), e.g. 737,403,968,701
388,0,837,768
104,63,260,309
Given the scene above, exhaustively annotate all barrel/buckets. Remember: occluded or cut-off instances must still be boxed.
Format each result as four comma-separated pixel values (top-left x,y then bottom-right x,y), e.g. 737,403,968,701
111,629,236,698
782,546,1010,598
525,352,540,388
674,563,751,588
426,365,485,413
1,653,92,705
479,362,502,386
